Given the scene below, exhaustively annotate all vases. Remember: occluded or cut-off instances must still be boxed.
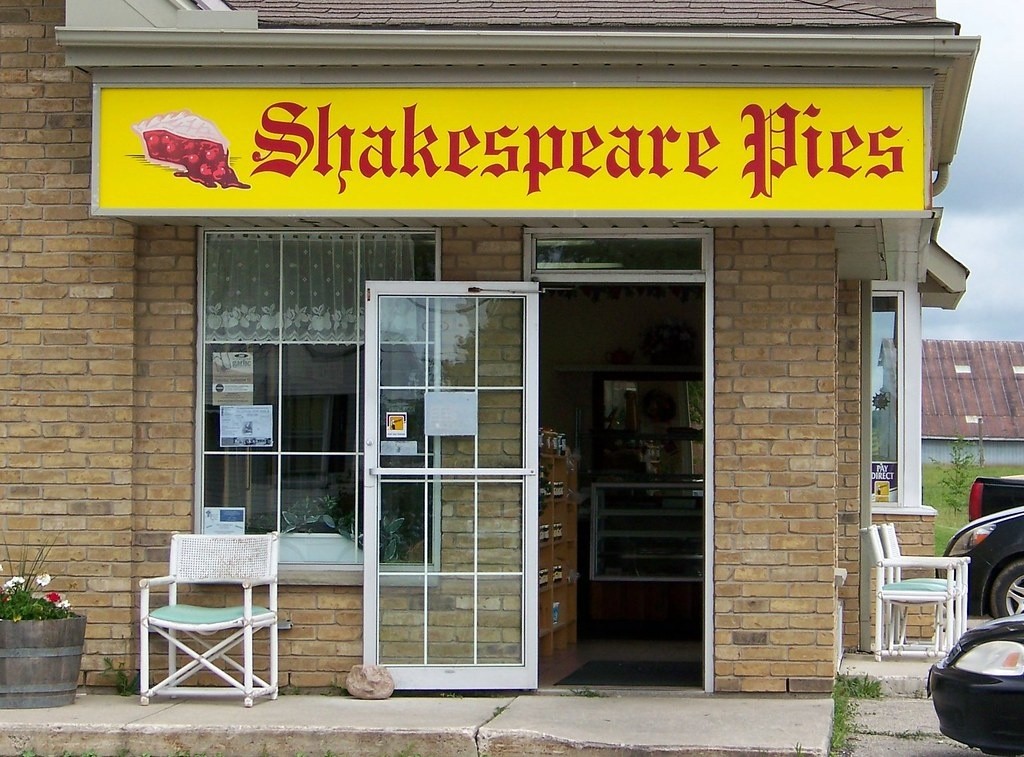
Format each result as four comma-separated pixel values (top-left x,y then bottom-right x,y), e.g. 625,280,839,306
0,615,87,709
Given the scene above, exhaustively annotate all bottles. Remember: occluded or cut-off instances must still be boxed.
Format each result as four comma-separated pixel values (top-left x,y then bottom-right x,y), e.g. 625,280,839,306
539,466,564,498
539,565,563,587
538,522,563,543
539,427,566,456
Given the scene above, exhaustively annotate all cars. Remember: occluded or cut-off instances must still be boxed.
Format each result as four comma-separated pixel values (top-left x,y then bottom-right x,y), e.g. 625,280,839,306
926,613,1024,757
935,505,1024,619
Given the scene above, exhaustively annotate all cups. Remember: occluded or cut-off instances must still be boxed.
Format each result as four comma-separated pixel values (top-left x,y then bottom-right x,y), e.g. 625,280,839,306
552,602,560,625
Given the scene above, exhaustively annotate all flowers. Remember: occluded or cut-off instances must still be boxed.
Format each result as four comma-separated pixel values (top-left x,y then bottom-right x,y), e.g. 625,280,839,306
0,527,73,622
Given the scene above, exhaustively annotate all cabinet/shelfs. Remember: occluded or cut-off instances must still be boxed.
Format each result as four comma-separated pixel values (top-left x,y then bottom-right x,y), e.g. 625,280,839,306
538,452,577,656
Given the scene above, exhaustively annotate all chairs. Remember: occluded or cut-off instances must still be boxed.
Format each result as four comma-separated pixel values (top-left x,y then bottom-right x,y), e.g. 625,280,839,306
859,523,971,662
138,530,278,707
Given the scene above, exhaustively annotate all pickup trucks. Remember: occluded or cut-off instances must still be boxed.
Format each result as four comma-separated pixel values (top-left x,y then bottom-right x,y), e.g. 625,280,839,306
966,475,1024,523
